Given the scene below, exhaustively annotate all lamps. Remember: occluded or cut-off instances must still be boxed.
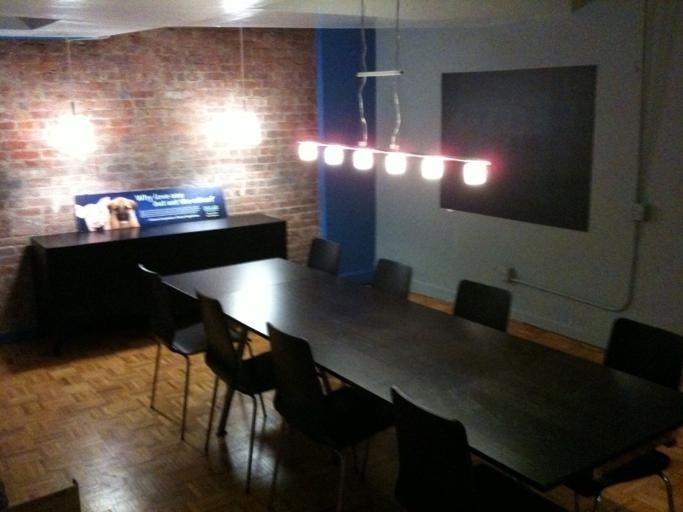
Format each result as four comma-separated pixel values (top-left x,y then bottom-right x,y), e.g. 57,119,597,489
295,0,494,187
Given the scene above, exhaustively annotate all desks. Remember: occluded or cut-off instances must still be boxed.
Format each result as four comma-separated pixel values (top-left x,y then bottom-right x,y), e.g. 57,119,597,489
162,257,680,510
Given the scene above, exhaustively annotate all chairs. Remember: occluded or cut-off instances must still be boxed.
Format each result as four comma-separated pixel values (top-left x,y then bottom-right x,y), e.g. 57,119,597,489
370,258,413,300
138,263,269,440
306,238,341,276
389,385,557,510
452,280,513,333
264,321,402,510
561,317,680,510
196,289,313,493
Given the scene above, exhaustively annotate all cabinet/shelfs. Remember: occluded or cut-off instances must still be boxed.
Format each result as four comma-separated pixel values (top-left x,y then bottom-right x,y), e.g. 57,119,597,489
13,211,289,356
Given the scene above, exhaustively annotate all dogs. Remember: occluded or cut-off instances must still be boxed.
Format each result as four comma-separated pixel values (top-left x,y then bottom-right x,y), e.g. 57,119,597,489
106,197,140,231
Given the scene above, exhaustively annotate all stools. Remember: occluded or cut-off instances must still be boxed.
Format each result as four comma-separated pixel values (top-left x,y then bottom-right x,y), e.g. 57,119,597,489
1,443,82,511
